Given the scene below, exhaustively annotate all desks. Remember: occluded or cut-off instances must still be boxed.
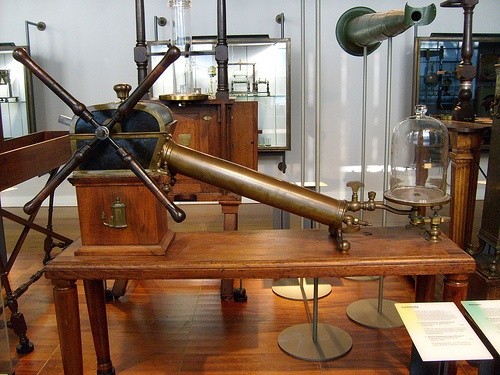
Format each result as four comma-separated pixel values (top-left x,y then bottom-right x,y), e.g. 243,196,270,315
41,225,476,374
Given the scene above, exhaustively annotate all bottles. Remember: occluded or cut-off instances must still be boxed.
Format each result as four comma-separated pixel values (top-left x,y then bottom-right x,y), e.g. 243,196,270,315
390,104,451,204
